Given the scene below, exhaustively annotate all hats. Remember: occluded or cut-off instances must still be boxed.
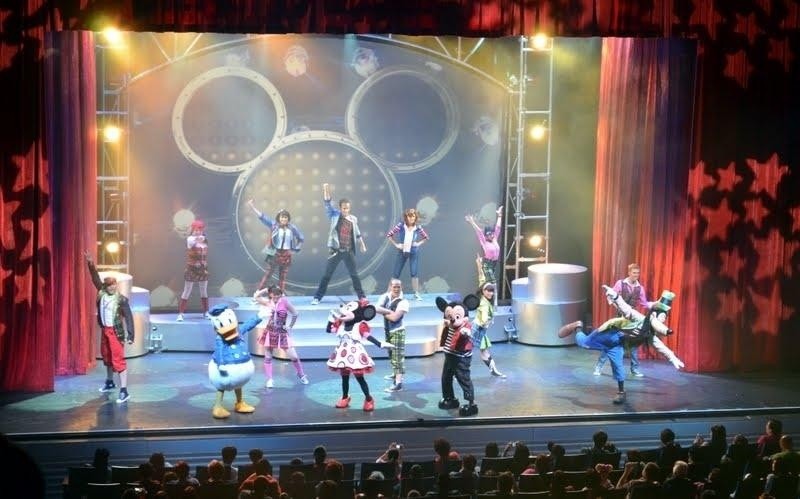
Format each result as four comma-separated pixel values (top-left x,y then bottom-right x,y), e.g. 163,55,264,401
191,220,205,231
100,277,119,288
485,225,496,236
651,288,677,313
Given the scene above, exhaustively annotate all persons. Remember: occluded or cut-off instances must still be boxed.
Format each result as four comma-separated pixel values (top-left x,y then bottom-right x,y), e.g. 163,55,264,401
458,251,507,379
311,183,370,306
83,248,135,404
356,418,800,499
373,277,410,393
465,205,505,306
246,198,304,302
594,263,648,379
558,284,685,405
83,446,344,499
175,221,215,323
253,285,310,389
385,208,431,302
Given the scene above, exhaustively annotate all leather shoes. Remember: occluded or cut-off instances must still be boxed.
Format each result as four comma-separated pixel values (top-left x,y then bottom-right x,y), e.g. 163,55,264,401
459,403,479,416
437,396,461,409
556,319,582,341
612,391,629,403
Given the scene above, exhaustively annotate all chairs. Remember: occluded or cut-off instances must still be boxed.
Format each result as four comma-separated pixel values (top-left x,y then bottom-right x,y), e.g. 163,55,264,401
444,459,465,472
660,447,688,466
357,479,397,493
762,472,800,498
198,485,235,498
481,457,514,474
402,461,436,476
360,462,399,478
450,493,470,497
88,483,120,499
317,463,355,481
195,466,210,479
69,467,98,498
563,490,587,499
121,483,137,492
728,445,757,462
451,477,466,487
111,466,142,483
761,444,783,456
581,451,620,471
279,464,313,483
600,488,626,498
733,479,760,498
162,467,174,474
402,478,437,494
743,462,768,479
239,465,274,479
516,490,551,498
530,456,536,465
662,478,693,499
520,473,553,492
479,494,497,498
691,447,724,461
245,483,279,493
563,470,589,488
561,453,589,472
690,462,713,482
627,482,661,498
480,476,501,488
166,482,198,499
608,469,623,484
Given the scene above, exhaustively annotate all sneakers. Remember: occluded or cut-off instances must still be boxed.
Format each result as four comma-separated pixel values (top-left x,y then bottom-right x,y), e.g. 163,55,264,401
97,382,117,394
202,311,210,319
310,298,320,305
593,367,601,376
175,313,186,325
630,371,644,378
264,379,274,388
413,292,422,301
489,367,508,381
384,380,404,394
295,372,309,386
114,391,132,405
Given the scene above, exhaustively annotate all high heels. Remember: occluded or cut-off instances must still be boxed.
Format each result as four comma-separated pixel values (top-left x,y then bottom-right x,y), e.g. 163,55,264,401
362,396,375,412
335,393,351,408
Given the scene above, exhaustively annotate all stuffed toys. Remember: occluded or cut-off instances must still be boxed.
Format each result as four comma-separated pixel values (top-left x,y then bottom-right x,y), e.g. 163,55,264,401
205,301,274,420
434,293,481,416
326,297,398,412
